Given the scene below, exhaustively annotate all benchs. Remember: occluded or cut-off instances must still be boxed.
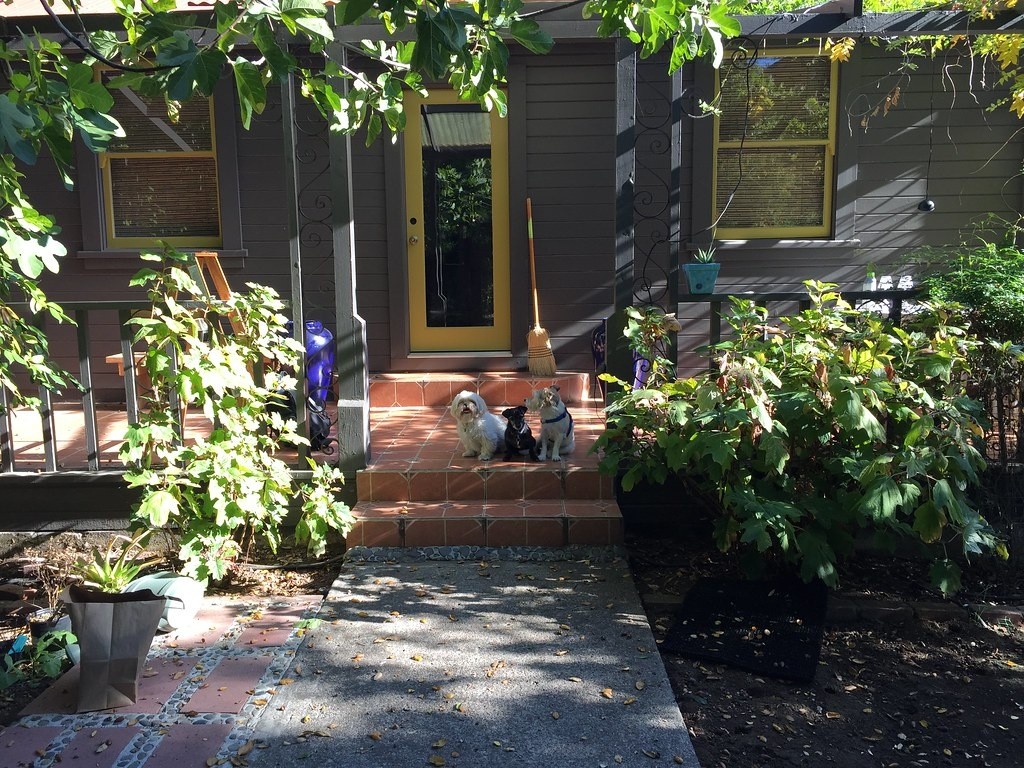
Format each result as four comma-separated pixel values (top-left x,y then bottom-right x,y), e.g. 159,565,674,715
106,351,270,409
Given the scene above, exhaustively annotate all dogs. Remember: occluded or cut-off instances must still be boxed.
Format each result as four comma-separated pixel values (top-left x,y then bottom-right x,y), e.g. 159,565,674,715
450,390,507,461
502,406,540,462
523,385,576,462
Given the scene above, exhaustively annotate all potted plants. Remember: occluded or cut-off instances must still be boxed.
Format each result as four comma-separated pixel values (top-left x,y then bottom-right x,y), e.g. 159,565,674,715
682,228,721,294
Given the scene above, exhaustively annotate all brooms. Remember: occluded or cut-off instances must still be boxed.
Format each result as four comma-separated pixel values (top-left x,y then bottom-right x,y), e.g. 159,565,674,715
524,197,558,378
